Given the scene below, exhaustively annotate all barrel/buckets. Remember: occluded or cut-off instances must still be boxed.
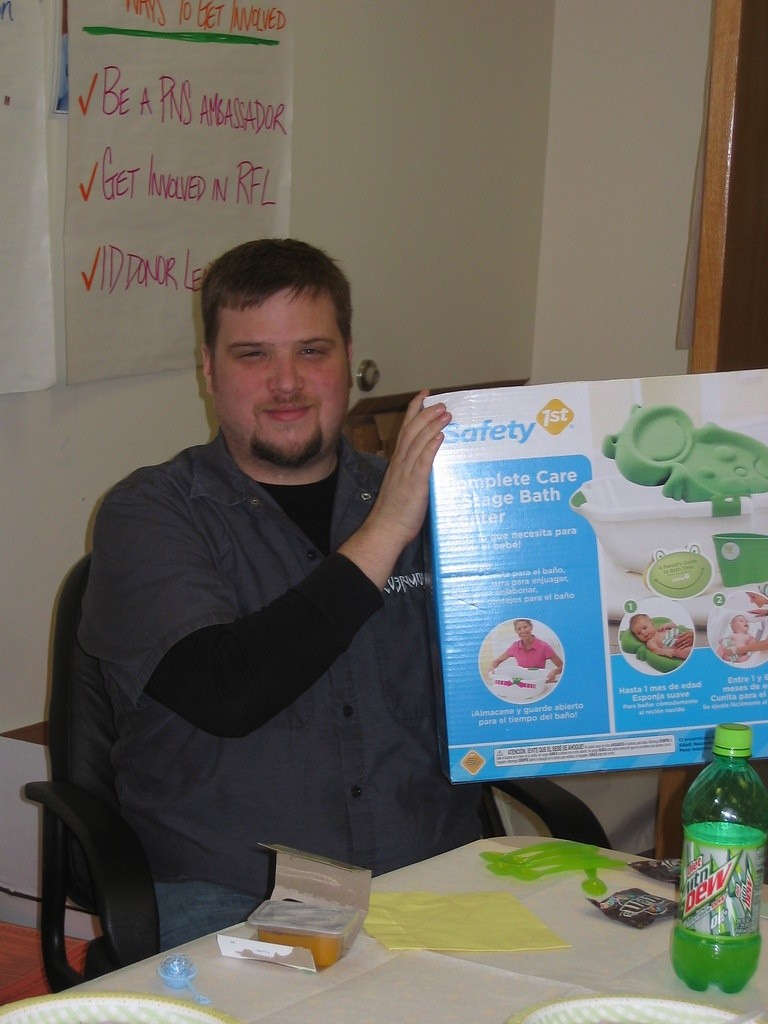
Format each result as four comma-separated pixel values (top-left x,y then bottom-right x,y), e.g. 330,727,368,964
711,532,768,589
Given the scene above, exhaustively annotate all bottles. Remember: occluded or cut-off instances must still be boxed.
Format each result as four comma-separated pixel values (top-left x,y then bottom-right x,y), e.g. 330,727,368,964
671,723,768,995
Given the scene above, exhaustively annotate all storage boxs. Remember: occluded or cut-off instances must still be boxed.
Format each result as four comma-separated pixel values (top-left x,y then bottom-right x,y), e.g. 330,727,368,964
423,366,767,787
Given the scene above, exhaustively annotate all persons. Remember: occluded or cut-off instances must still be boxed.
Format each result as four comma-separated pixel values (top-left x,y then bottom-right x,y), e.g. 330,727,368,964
630,613,694,661
716,593,768,662
488,621,564,683
76,237,495,953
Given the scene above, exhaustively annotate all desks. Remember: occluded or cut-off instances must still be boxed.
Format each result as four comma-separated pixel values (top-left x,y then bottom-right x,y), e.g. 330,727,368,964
0,835,768,1024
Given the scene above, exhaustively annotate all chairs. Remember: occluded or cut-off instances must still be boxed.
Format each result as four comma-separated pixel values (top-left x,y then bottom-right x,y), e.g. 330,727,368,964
24,554,617,994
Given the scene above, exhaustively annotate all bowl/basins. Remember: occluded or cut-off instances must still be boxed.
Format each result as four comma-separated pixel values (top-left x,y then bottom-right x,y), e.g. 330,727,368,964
248,900,355,967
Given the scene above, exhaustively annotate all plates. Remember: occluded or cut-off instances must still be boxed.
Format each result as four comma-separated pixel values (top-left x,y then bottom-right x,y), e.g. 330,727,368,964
1,992,249,1023
502,992,744,1023
